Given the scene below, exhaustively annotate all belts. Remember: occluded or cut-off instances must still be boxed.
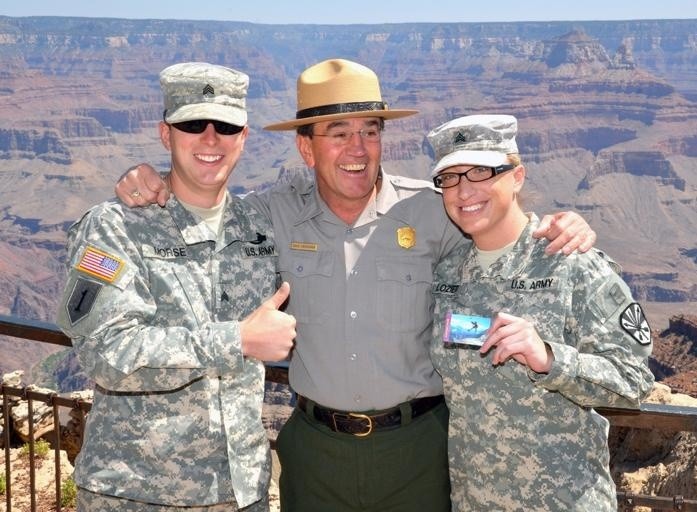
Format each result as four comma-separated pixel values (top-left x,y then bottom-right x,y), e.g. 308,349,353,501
290,389,447,438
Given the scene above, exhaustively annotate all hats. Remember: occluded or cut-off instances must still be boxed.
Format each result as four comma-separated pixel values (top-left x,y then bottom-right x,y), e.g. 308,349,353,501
261,56,421,137
422,109,521,180
158,61,251,128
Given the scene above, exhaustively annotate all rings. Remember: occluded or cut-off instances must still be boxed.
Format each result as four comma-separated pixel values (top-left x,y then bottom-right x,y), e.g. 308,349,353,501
130,189,140,197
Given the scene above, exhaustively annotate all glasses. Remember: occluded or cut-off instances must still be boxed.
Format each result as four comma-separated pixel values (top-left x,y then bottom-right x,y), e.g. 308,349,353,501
313,129,384,146
431,162,515,189
169,111,242,137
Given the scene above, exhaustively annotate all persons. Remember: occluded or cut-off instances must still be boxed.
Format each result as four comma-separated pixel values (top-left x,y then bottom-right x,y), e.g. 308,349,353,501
46,57,296,512
114,58,596,512
423,112,657,512
